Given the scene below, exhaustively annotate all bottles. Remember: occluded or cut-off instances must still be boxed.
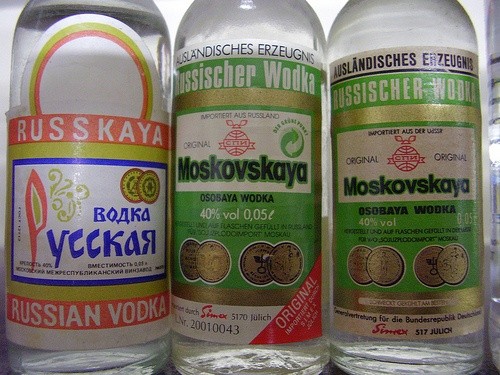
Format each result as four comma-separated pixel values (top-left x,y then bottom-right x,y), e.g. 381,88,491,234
172,0,332,375
486,1,500,373
326,1,487,374
4,0,172,375
